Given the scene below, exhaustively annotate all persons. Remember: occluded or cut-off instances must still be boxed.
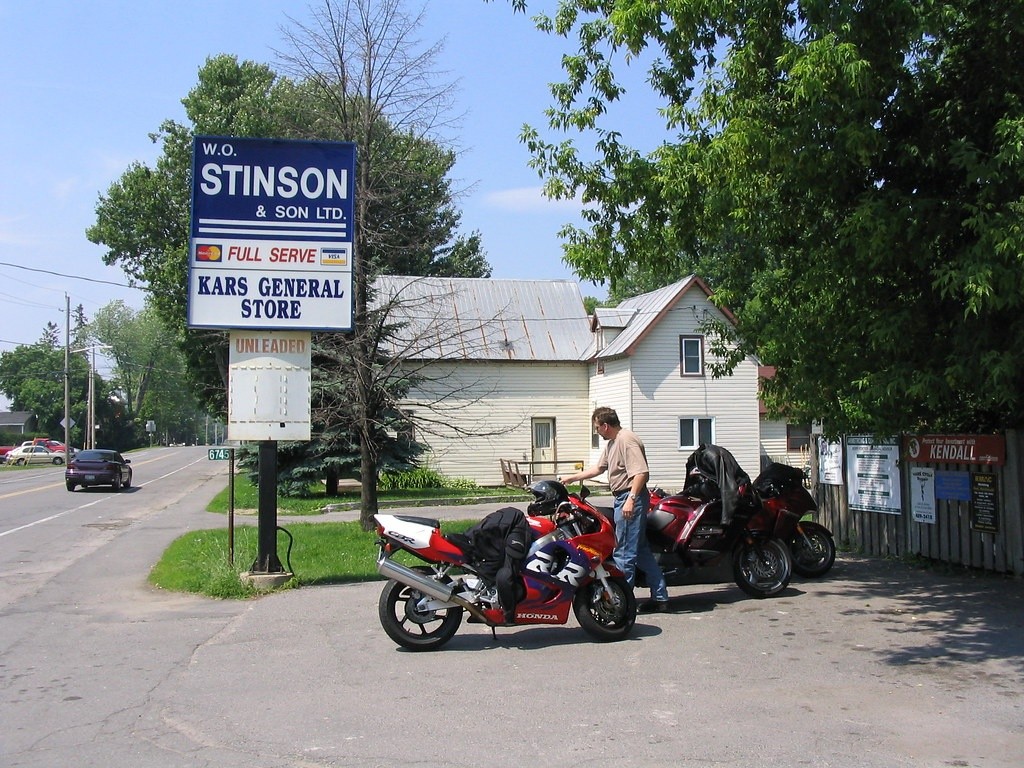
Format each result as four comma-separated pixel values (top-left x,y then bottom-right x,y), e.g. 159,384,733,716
559,407,670,613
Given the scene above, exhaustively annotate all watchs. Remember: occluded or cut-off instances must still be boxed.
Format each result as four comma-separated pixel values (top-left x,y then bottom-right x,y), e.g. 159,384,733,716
629,494,636,500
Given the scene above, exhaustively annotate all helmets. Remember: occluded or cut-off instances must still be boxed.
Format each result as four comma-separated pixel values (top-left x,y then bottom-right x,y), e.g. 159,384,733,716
526,479,572,516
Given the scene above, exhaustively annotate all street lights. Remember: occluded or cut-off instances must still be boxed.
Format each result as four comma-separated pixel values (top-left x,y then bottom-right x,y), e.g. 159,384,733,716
64,345,114,468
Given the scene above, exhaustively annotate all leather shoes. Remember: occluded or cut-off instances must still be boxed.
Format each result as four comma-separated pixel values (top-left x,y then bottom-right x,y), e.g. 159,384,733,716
637,598,673,613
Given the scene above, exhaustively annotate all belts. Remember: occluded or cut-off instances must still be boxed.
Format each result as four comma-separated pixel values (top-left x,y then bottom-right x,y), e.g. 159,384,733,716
612,487,631,496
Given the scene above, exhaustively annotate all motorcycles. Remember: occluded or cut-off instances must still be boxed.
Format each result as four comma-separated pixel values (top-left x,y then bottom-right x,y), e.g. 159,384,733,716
741,467,837,578
373,477,638,651
589,445,794,599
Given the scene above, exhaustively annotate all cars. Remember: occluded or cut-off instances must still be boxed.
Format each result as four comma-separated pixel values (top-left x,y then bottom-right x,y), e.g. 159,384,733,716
3,445,73,465
64,449,133,492
0,439,83,463
